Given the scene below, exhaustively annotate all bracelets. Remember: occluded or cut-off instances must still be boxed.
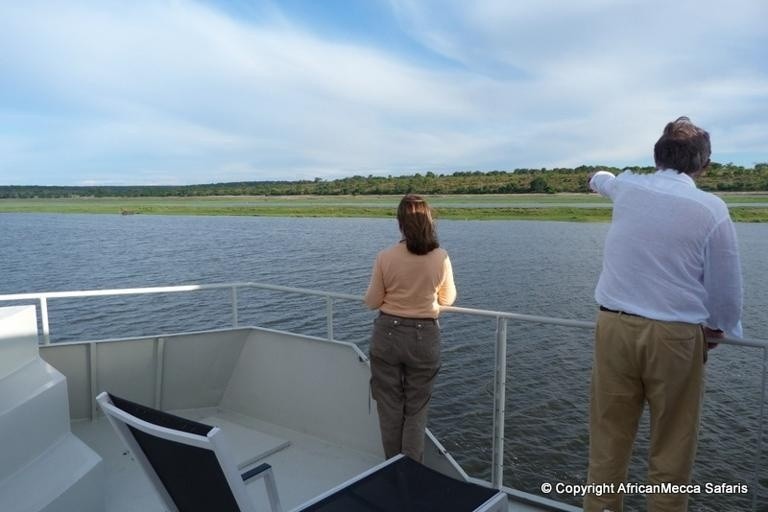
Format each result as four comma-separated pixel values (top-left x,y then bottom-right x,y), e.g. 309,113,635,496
709,328,723,333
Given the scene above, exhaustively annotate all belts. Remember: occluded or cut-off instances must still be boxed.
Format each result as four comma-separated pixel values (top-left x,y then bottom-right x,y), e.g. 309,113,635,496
600,305,637,316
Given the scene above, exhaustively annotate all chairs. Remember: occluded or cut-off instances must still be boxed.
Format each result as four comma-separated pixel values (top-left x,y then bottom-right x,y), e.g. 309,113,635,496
95,391,509,512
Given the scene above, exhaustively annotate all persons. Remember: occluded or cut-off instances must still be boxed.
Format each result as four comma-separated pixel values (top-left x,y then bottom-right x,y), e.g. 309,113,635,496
362,192,461,466
583,114,743,511
